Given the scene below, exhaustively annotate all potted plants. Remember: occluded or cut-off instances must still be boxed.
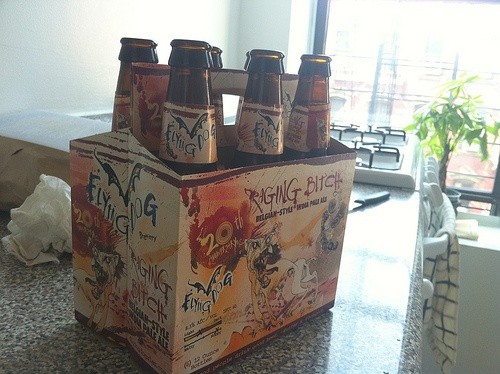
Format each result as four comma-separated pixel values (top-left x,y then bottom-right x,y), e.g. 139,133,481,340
402,70,500,220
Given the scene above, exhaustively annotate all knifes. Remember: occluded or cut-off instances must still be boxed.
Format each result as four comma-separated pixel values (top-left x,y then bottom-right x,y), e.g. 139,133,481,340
348,189,391,213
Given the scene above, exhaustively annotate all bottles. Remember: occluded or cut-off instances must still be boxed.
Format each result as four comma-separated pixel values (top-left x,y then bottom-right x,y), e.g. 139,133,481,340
231,48,287,169
112,37,160,139
285,54,332,160
157,38,225,176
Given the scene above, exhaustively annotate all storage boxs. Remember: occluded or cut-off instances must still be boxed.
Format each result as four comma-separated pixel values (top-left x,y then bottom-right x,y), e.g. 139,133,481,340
1,110,357,374
